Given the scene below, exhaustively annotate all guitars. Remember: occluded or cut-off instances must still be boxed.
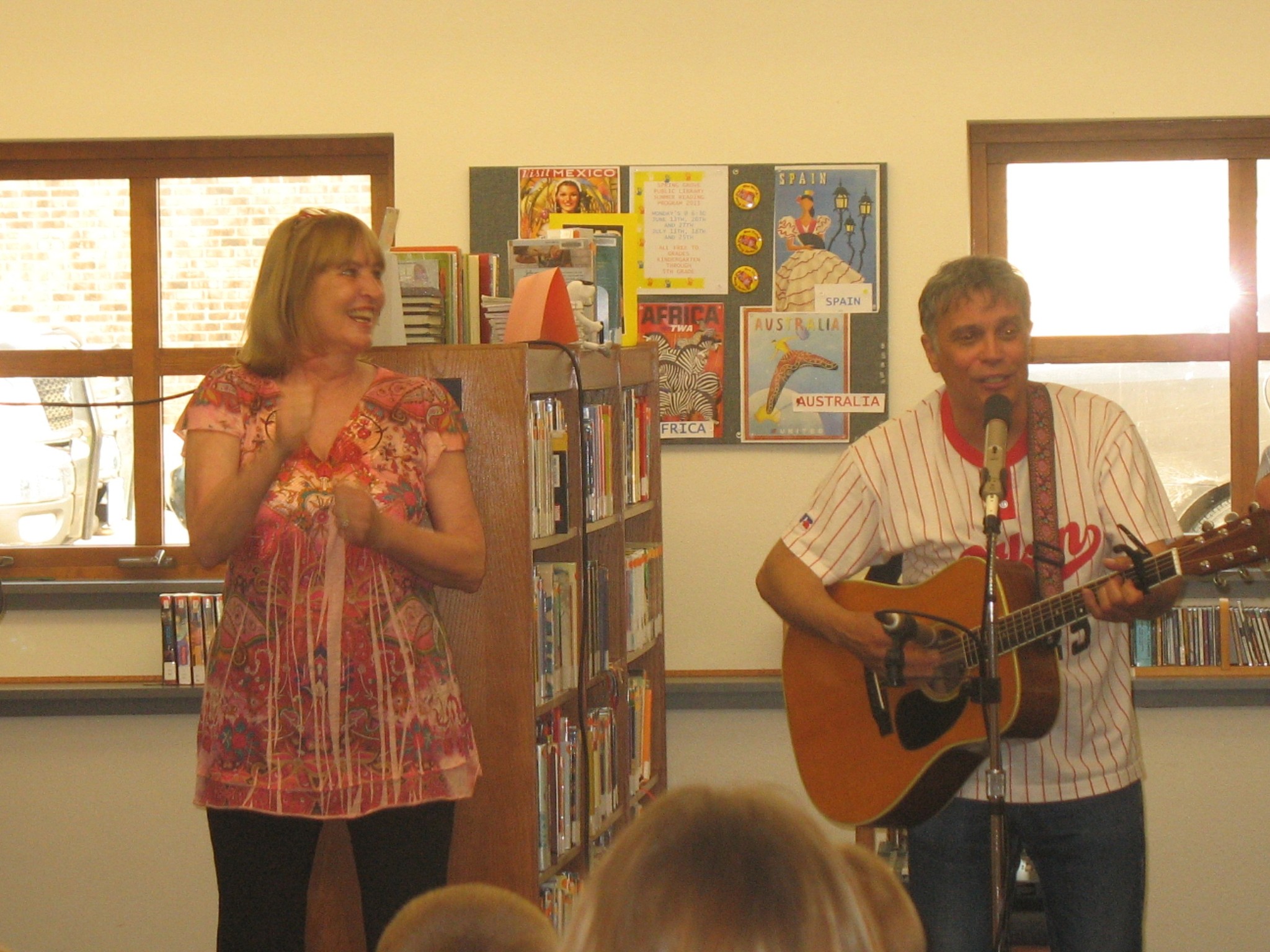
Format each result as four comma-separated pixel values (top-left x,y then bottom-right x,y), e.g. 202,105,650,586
782,502,1270,831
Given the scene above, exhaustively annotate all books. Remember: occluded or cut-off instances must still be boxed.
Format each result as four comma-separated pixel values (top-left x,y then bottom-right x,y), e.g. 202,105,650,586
380,205,637,353
528,660,658,943
159,588,224,687
518,390,666,539
518,532,667,706
1127,604,1270,668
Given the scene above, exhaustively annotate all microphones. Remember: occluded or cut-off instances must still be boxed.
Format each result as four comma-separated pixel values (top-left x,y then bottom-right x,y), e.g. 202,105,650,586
982,391,1014,534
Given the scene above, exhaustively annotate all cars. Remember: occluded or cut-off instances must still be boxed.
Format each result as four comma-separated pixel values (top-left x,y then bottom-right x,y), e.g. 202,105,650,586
0,376,132,550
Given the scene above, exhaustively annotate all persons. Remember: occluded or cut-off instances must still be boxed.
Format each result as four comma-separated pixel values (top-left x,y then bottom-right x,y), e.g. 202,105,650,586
755,256,1184,951
561,774,926,952
173,207,486,952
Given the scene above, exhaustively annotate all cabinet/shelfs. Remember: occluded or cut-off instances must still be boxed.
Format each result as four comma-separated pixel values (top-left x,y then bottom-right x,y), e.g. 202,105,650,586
304,339,664,952
0,579,228,715
1129,567,1269,694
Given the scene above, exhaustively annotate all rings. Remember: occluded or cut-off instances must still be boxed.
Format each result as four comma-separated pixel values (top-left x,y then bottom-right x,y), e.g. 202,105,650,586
338,516,350,531
